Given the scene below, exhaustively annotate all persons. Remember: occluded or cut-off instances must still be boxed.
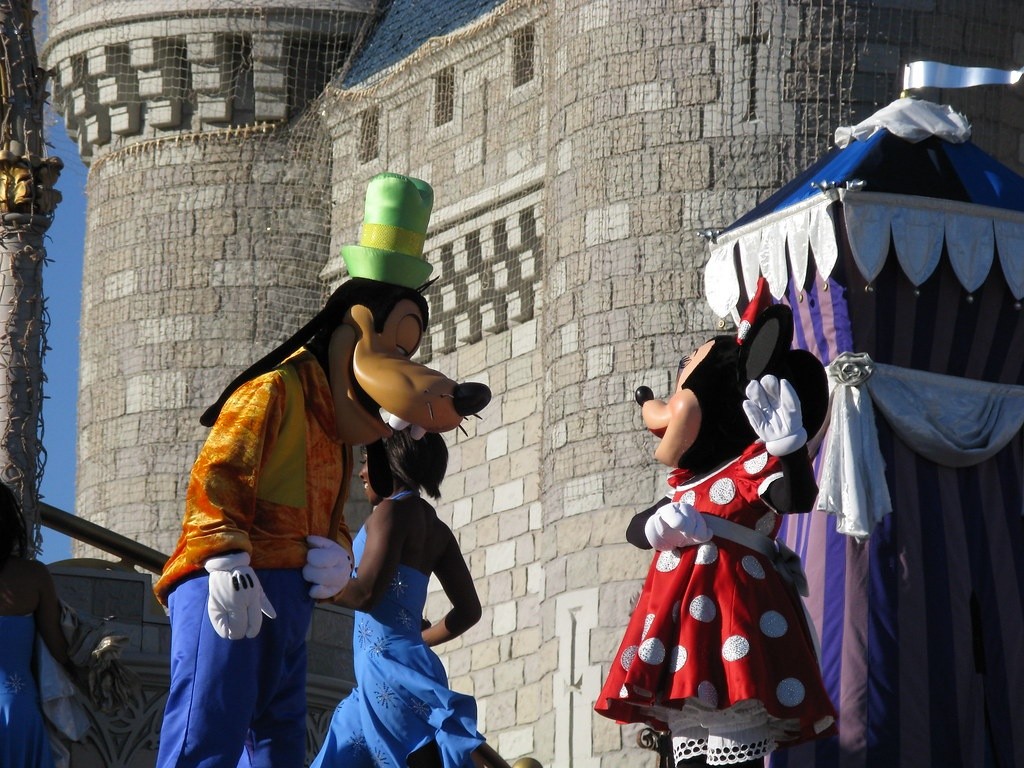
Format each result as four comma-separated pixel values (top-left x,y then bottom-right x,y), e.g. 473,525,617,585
0,481,90,768
309,428,542,768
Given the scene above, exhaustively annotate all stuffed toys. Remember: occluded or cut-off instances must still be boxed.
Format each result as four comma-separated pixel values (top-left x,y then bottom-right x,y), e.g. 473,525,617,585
596,279,837,768
152,173,491,768
